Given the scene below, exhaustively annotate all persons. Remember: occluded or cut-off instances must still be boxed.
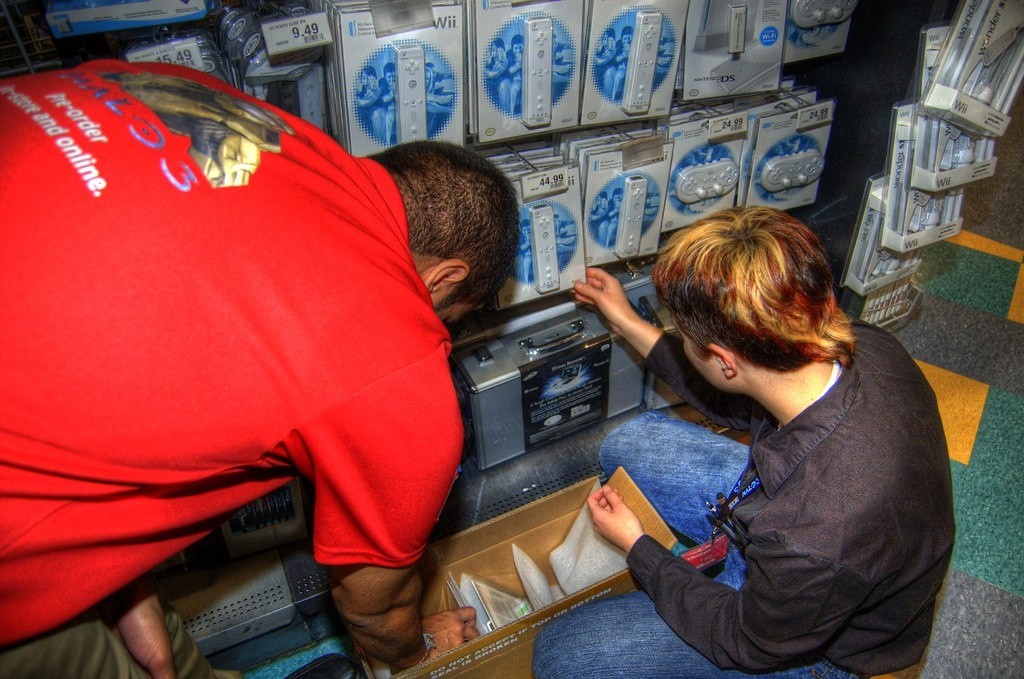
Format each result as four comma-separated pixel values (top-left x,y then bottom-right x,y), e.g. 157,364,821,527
532,204,956,679
0,60,521,679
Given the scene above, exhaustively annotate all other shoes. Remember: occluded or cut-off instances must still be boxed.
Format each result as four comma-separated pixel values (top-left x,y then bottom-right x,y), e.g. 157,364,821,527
283,653,361,679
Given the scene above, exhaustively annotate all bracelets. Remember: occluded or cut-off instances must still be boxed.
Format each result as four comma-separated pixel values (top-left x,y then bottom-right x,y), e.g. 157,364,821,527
420,631,436,665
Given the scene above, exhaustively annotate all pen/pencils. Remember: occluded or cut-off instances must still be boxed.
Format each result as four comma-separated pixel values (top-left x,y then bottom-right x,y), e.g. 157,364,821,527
705,492,739,541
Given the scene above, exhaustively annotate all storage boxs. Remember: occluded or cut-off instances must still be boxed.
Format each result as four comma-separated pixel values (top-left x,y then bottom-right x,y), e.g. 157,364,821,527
352,466,678,679
